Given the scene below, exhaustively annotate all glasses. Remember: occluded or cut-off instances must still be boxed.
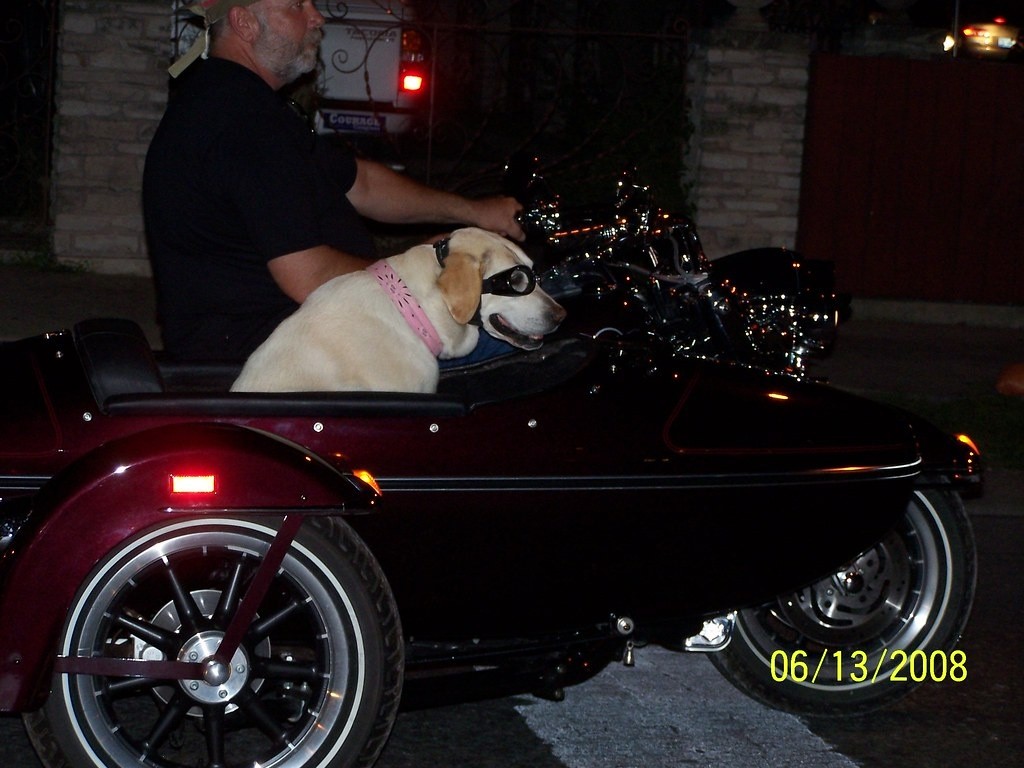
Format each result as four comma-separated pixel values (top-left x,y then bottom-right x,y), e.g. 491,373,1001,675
479,263,540,298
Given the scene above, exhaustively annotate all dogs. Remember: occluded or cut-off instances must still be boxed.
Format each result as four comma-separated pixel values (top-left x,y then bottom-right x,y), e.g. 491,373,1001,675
227,228,568,394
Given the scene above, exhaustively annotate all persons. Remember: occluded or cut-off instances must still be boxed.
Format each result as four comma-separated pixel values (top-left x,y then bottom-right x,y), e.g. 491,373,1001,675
141,0,525,369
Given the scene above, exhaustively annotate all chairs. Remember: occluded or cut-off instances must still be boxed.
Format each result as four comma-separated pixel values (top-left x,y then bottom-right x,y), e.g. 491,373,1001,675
72,318,164,412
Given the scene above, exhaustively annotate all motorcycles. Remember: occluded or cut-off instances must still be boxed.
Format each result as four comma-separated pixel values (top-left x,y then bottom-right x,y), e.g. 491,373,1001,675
0,151,983,768
